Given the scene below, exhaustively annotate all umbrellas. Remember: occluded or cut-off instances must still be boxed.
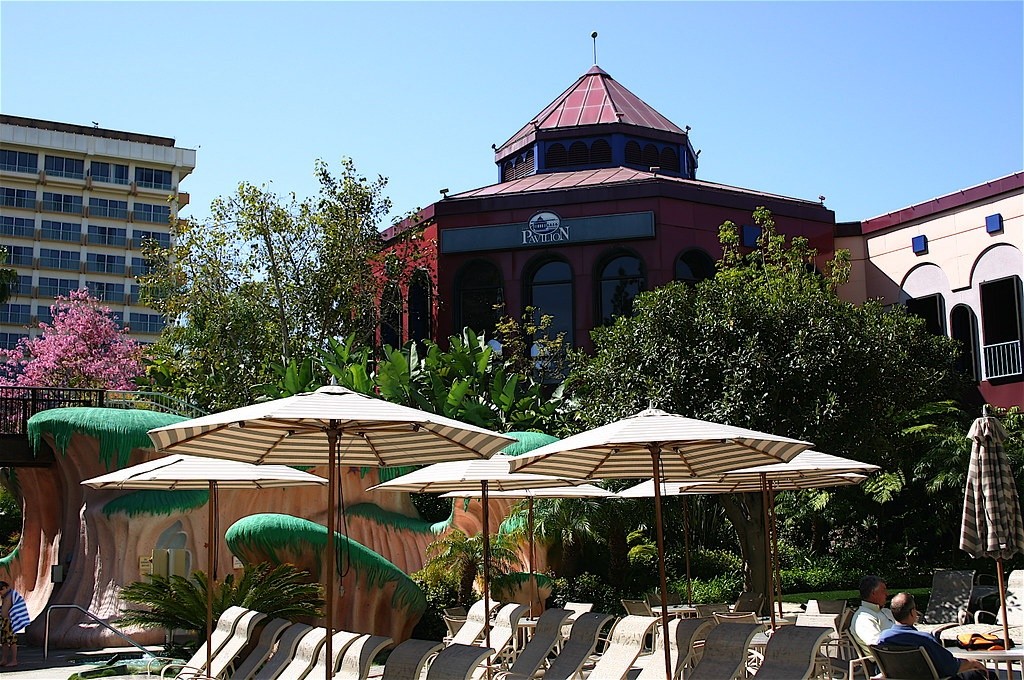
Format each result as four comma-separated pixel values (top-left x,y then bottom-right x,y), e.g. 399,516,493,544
958,404,1024,680
365,454,621,680
508,401,815,680
620,448,882,630
78,450,329,678
145,374,518,680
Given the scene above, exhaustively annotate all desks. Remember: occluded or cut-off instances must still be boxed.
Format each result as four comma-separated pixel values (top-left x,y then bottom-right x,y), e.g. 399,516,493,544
944,647,1024,680
651,603,697,617
756,616,797,626
749,632,832,645
488,617,574,627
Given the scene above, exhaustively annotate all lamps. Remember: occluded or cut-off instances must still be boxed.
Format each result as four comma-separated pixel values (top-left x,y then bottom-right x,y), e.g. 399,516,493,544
492,144,498,152
686,125,691,135
441,189,449,200
818,194,825,205
615,112,624,123
650,167,661,178
529,119,539,130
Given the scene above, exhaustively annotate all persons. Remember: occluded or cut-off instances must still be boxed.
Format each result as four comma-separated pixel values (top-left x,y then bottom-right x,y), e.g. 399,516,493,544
0,581,31,667
849,575,897,676
877,591,999,680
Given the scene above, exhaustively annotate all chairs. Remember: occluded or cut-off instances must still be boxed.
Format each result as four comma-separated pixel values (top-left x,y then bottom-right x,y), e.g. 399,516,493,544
147,568,1024,680
